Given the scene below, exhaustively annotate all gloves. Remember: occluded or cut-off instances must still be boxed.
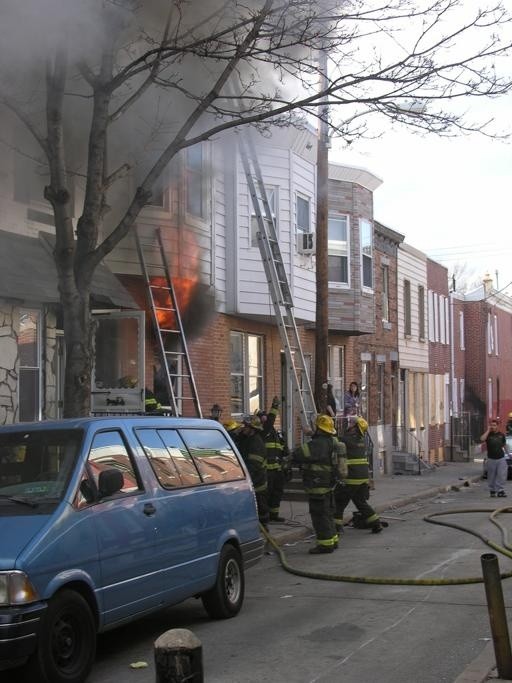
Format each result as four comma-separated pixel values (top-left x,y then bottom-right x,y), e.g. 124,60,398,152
272,396,279,408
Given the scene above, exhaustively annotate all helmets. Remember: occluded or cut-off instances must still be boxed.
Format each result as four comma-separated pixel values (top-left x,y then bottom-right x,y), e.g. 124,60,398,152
243,412,263,431
120,376,137,388
219,419,241,432
355,417,369,436
313,414,338,435
253,408,265,419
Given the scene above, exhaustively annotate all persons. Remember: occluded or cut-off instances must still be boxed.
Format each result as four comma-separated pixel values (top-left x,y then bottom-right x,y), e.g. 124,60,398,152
88,375,165,415
286,412,343,555
205,378,385,538
479,419,512,497
506,411,511,435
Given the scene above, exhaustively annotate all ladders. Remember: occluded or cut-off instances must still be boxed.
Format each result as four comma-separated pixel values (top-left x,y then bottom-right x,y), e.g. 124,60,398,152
133,225,204,418
224,72,318,432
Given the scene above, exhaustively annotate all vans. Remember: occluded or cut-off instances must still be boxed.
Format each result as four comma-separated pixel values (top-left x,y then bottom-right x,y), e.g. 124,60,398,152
1,417,261,677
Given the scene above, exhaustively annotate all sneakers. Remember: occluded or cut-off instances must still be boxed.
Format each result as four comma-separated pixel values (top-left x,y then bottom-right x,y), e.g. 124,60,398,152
490,491,497,497
499,490,507,496
309,545,334,553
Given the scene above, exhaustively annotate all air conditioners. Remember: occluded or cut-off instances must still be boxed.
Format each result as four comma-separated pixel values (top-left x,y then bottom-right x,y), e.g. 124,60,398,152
297,232,316,254
